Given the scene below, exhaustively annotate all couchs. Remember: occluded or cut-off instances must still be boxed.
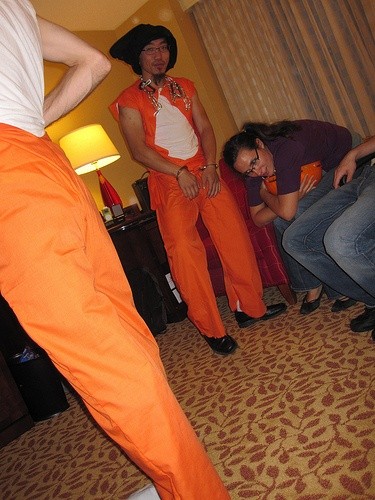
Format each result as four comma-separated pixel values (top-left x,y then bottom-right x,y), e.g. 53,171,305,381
195,158,298,305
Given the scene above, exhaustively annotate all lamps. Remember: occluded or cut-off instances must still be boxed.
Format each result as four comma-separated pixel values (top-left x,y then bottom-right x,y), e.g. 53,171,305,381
58,122,128,223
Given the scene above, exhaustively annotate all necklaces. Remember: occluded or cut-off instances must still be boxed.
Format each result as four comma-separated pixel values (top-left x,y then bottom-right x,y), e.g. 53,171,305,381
259,163,278,187
139,74,185,111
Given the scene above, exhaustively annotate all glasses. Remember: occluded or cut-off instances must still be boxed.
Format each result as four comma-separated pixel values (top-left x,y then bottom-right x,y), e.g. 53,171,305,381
140,43,171,56
240,147,261,178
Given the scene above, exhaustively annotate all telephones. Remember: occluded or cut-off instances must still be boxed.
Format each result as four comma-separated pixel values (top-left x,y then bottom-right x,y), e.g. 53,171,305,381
101,207,115,227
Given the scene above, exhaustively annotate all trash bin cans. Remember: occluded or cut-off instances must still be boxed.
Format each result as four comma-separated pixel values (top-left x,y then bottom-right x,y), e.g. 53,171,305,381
7,347,70,422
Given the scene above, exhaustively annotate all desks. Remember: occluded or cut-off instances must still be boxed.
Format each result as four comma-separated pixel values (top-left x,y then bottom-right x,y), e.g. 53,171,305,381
106,210,188,335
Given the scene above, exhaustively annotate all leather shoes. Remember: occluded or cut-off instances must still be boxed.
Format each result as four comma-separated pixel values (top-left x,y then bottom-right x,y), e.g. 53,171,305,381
331,296,357,313
235,303,287,327
299,286,324,314
204,335,236,355
350,307,375,332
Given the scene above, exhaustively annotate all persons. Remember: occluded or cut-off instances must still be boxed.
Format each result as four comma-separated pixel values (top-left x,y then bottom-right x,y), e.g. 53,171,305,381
222,119,366,315
107,23,288,355
0,1,232,500
282,136,375,340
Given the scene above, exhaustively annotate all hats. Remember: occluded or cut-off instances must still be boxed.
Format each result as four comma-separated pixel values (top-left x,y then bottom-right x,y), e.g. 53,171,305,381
110,24,178,75
